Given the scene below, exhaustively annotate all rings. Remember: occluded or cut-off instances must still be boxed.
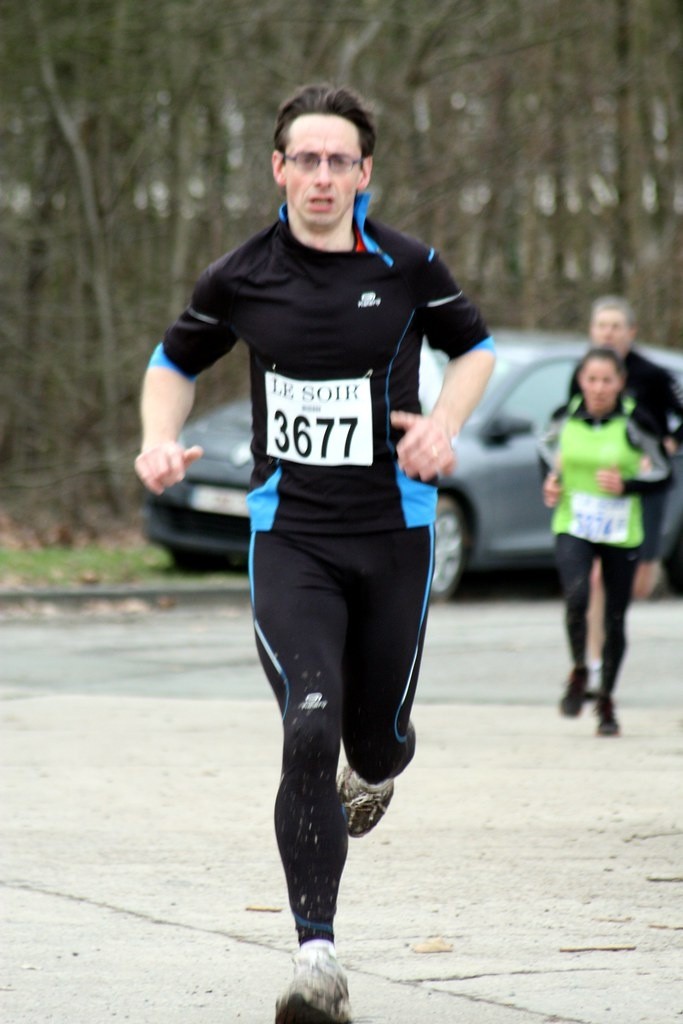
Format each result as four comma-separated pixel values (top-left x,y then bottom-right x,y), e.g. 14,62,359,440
432,445,438,456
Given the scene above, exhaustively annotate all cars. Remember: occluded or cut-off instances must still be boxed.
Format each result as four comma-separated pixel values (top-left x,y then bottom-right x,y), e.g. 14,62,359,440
137,328,683,607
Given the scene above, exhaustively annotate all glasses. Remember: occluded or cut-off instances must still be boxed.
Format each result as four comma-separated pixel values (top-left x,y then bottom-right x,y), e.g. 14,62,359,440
284,151,364,175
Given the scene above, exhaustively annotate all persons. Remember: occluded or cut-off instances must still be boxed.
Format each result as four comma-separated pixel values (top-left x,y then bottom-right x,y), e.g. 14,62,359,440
569,294,683,695
133,82,498,1024
544,349,675,736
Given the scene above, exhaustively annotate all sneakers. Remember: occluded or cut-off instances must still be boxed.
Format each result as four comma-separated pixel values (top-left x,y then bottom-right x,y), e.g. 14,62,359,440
274,945,352,1024
334,760,393,839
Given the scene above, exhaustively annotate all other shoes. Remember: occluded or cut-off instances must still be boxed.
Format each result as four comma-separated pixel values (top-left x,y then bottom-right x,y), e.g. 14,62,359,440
585,661,601,691
560,667,589,716
595,697,620,734
